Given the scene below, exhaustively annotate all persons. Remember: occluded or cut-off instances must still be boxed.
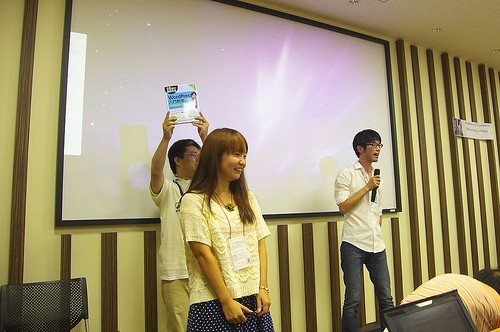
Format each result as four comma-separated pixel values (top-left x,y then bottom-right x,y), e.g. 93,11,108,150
179,128,275,331
382,268,500,332
189,92,198,108
149,110,209,332
334,129,394,332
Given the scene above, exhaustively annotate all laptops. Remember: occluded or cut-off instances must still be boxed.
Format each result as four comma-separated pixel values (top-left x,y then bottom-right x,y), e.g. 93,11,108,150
383,289,479,332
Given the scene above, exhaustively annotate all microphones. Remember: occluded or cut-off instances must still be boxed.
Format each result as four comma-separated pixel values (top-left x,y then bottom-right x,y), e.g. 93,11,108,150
371,169,380,202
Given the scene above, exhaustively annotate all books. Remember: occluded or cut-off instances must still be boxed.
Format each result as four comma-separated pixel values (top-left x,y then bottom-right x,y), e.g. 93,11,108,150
165,83,200,124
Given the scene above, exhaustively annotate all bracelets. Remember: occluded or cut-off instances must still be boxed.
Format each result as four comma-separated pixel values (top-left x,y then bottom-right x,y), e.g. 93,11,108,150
259,286,269,293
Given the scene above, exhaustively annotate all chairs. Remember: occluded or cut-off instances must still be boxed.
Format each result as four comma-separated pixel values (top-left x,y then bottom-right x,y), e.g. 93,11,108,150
0,277,89,332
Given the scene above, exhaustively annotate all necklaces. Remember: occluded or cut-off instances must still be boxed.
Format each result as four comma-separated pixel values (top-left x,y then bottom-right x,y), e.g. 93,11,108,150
215,192,234,211
172,180,183,197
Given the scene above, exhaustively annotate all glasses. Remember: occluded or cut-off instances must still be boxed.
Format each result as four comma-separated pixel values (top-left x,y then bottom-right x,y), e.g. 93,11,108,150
183,152,200,158
365,142,383,148
